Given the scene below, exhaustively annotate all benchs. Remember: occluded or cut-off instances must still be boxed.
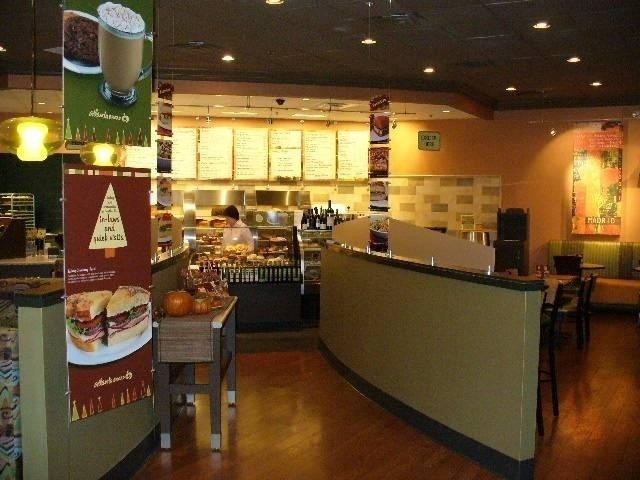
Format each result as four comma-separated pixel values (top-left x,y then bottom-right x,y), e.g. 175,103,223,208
548,240,640,312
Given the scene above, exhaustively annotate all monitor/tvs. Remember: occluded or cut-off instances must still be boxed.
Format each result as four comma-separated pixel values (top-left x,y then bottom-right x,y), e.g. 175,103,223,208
500,215,526,241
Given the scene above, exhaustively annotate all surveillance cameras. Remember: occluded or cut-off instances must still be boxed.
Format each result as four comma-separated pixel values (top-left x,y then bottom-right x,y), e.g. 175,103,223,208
276,99,285,105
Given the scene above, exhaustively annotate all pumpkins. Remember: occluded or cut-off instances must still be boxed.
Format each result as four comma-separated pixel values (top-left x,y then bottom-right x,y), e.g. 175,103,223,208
194,299,208,314
161,288,193,316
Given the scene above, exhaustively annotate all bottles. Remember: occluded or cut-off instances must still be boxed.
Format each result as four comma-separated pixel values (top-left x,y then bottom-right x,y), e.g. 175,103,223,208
199,260,300,283
301,200,351,229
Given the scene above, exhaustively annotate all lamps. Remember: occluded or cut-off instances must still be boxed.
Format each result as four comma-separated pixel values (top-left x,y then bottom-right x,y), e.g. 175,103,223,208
1,1,63,163
80,140,127,168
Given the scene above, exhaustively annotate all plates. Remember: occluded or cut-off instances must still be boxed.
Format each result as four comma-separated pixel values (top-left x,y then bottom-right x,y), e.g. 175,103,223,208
62,9,103,75
66,301,152,366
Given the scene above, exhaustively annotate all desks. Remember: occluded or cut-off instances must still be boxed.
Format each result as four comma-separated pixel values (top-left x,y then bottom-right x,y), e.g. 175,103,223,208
152,295,238,453
582,264,604,269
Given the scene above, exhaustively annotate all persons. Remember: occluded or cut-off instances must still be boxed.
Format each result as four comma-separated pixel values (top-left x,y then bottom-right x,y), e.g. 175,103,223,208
222,205,254,252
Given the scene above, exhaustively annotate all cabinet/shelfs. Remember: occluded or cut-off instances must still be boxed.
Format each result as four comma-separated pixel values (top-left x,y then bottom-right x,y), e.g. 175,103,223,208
302,229,334,330
184,226,302,332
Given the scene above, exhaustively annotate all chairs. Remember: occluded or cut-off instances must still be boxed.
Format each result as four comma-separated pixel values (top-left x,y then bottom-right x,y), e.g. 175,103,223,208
540,253,600,437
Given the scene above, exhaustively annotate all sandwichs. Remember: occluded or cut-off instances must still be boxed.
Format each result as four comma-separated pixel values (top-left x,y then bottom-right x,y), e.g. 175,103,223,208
67,290,112,351
106,286,151,347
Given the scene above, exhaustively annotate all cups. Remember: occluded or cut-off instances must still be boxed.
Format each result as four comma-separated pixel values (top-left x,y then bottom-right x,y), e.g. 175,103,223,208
98,4,153,107
